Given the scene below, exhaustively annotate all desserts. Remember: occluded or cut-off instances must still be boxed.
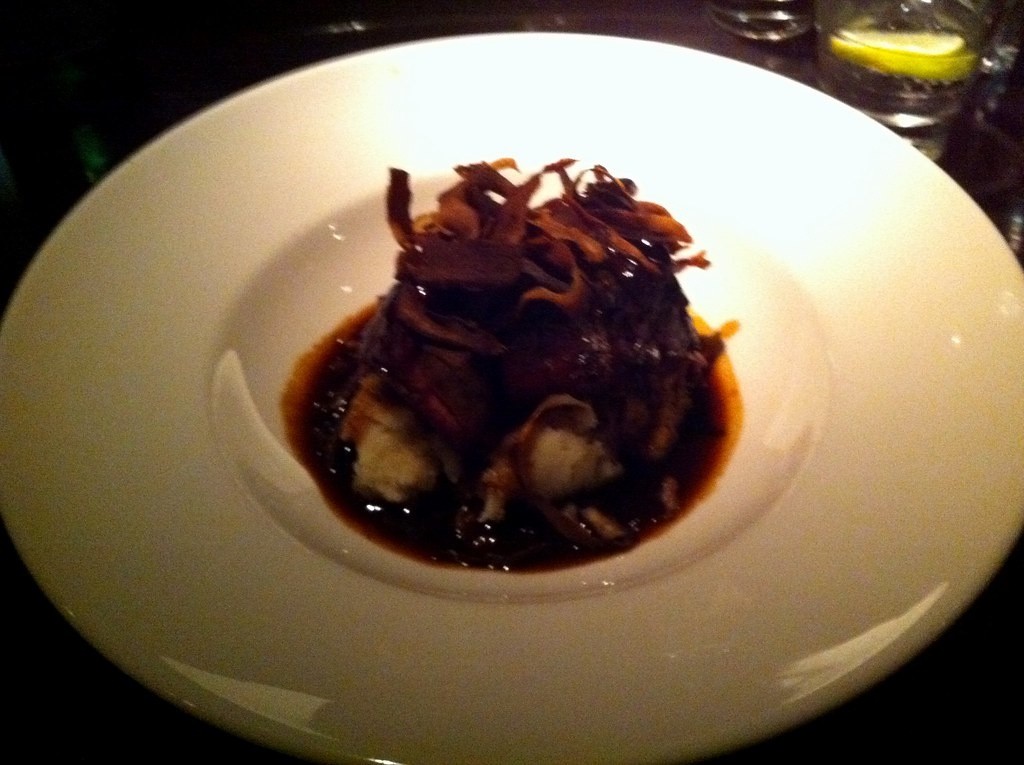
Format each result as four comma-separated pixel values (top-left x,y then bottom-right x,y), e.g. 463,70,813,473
280,155,741,576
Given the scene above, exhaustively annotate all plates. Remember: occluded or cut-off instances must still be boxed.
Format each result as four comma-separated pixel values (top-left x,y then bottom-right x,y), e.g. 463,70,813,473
0,28,1024,765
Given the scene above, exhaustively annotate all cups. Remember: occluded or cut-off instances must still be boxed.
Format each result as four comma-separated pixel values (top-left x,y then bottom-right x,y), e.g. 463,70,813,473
705,0,813,40
813,0,995,164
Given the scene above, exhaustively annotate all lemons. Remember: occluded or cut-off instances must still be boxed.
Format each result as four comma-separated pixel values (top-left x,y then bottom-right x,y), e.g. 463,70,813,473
829,30,979,83
